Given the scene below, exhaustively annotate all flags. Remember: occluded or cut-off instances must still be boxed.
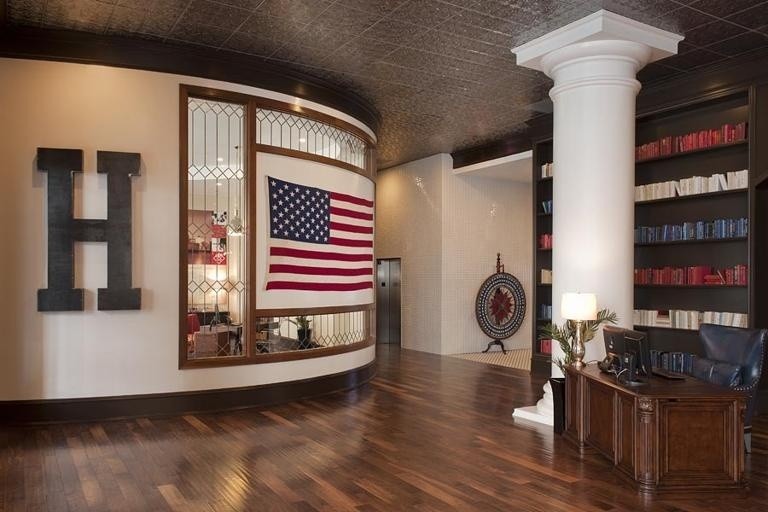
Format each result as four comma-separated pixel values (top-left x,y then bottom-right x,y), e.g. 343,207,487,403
262,174,373,291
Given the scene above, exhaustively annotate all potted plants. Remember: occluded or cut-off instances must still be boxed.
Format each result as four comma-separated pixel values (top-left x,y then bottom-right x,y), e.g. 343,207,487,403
287,315,313,349
535,307,619,435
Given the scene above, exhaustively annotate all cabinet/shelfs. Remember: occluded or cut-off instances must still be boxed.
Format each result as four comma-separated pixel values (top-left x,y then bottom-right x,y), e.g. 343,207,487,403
524,76,754,378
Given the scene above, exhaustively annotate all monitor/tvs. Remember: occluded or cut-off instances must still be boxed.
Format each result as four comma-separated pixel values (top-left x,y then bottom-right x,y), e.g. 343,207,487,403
618,328,650,386
603,326,628,372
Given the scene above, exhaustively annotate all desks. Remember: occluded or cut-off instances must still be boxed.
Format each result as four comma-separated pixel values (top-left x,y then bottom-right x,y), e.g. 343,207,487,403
559,361,749,491
199,321,279,355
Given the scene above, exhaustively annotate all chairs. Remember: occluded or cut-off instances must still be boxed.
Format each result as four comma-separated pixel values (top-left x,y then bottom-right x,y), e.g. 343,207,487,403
694,323,767,454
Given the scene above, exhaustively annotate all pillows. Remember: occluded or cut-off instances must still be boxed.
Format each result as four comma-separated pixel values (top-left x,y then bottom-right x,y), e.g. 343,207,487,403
692,355,744,387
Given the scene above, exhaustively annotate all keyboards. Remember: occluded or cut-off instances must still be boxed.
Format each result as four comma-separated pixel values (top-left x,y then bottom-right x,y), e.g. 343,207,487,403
651,368,686,380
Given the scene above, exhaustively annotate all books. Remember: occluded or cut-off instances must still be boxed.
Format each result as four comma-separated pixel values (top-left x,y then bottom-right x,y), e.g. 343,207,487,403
539,162,554,355
633,121,748,374
